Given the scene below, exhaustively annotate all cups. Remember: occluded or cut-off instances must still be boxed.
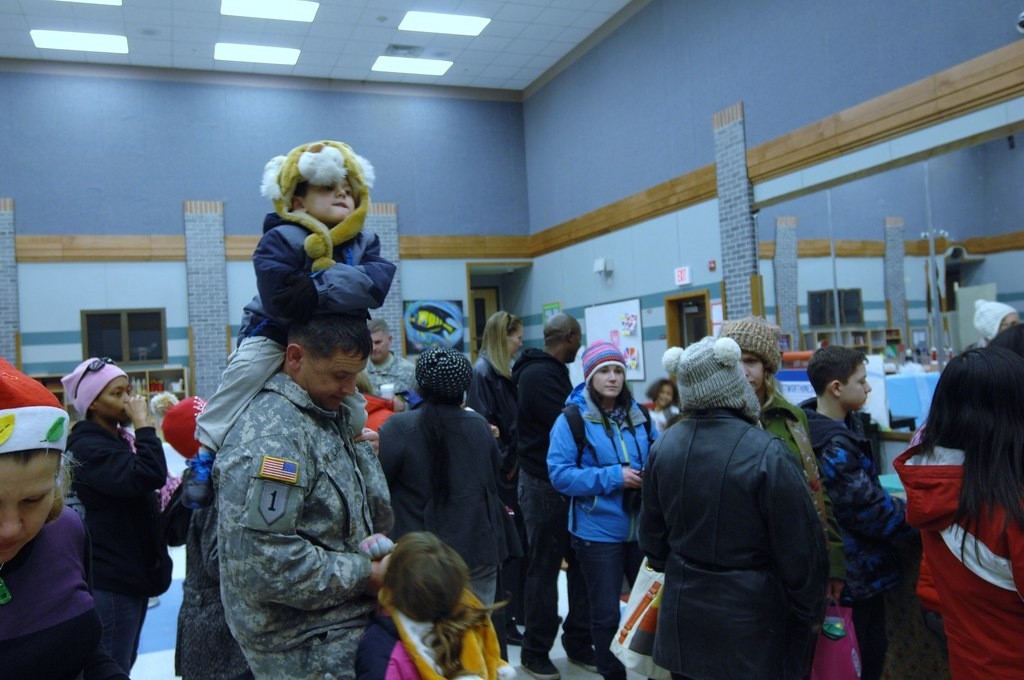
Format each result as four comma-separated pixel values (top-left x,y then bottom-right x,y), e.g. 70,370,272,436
380,384,395,401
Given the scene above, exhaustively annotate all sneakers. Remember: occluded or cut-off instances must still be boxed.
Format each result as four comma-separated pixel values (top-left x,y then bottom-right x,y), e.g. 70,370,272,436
181,453,214,509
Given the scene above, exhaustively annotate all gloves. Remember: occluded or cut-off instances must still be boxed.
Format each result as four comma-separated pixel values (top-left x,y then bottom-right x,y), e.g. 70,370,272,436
358,533,393,560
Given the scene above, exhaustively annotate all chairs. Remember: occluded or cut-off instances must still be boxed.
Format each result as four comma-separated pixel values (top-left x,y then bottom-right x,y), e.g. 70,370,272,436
888,407,917,432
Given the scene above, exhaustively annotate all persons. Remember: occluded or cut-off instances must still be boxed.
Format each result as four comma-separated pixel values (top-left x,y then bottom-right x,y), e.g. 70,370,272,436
0,300,1024,679
183,141,397,509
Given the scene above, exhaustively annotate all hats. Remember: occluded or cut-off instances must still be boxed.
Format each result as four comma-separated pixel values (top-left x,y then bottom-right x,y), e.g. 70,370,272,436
0,357,69,454
973,299,1017,341
150,390,208,460
260,140,376,274
662,316,783,433
416,348,473,403
580,340,626,387
61,357,128,420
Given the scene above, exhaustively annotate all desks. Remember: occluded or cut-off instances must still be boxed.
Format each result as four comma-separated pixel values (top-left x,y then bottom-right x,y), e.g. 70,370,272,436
886,372,941,434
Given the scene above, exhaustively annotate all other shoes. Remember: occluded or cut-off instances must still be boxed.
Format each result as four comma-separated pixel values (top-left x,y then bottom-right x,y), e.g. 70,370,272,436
505,505,515,517
504,625,523,646
567,647,599,673
520,655,560,680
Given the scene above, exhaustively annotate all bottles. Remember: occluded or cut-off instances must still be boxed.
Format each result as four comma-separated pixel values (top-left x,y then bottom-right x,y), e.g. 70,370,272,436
131,375,164,394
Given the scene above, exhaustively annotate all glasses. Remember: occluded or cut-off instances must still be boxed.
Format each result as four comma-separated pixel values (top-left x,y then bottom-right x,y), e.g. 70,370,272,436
74,357,115,399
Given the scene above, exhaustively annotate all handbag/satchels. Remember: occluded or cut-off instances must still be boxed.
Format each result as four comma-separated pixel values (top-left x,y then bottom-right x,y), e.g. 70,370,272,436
148,552,173,598
544,506,571,554
161,482,192,547
609,556,672,680
810,599,862,680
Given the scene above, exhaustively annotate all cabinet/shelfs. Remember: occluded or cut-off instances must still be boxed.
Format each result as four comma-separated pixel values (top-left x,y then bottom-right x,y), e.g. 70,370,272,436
28,368,188,441
777,327,901,361
